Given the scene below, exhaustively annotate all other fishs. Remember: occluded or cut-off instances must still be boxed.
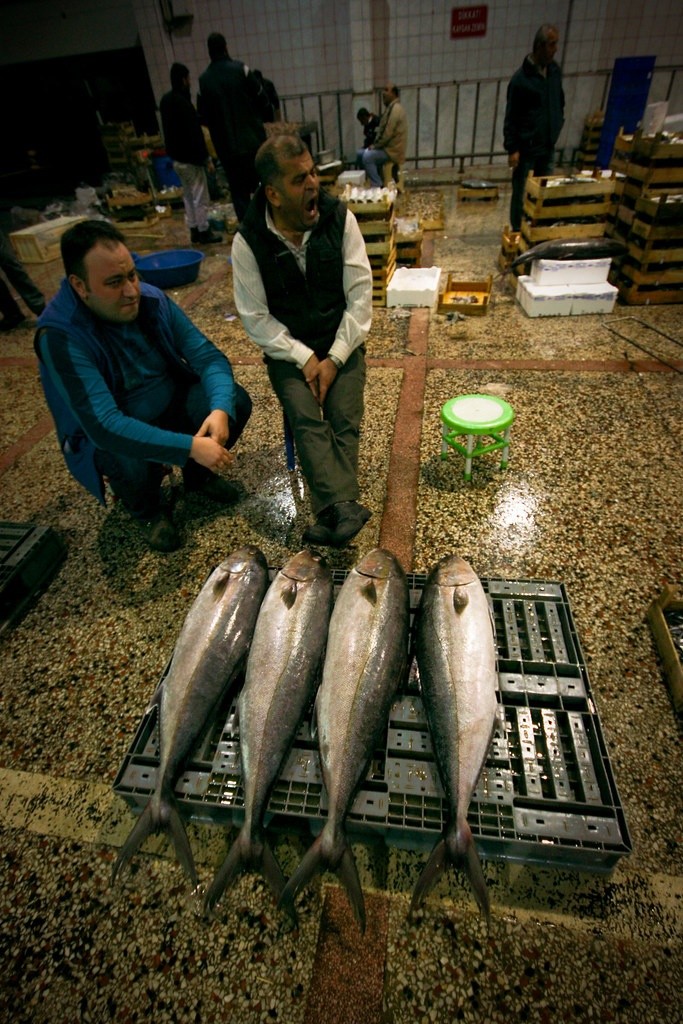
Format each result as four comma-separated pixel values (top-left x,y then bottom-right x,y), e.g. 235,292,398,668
411,554,497,930
199,547,334,933
110,544,271,897
494,239,630,283
275,548,412,939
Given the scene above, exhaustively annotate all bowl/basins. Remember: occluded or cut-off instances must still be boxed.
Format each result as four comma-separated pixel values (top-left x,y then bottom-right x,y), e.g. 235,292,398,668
317,149,335,164
133,248,205,291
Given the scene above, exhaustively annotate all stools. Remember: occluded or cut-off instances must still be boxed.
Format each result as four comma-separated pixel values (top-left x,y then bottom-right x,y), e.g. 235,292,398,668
442,394,515,481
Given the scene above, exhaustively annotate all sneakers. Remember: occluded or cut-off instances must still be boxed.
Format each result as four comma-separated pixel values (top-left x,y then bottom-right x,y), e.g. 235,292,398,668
332,502,373,543
303,515,337,545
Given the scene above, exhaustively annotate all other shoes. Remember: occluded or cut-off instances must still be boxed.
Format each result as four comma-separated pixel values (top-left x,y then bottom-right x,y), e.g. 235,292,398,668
127,507,180,552
183,474,239,503
199,229,223,244
190,226,201,243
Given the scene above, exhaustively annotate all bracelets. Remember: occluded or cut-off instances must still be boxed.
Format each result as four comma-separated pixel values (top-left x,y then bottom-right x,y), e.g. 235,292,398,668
328,355,342,368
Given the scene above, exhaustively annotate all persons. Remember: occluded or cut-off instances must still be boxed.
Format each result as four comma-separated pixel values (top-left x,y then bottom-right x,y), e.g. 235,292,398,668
355,84,408,186
199,32,280,222
32,220,252,552
0,226,47,330
503,23,565,233
231,133,373,547
160,62,223,244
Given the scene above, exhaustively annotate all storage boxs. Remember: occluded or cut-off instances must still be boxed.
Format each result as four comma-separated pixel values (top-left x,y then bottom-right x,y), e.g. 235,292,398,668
497,111,683,319
102,120,161,166
329,169,492,317
10,215,90,305
